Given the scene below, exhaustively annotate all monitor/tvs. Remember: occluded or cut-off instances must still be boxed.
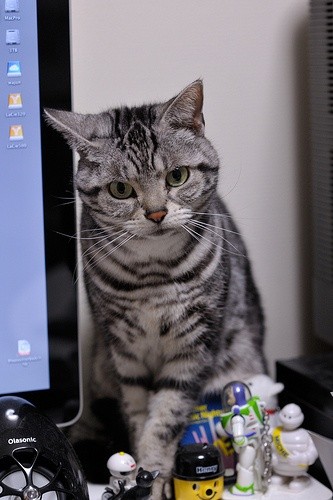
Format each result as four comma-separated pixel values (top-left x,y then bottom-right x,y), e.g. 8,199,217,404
0,0,83,430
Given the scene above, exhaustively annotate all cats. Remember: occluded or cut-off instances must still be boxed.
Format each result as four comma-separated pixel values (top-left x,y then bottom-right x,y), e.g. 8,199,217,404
42,78,272,500
